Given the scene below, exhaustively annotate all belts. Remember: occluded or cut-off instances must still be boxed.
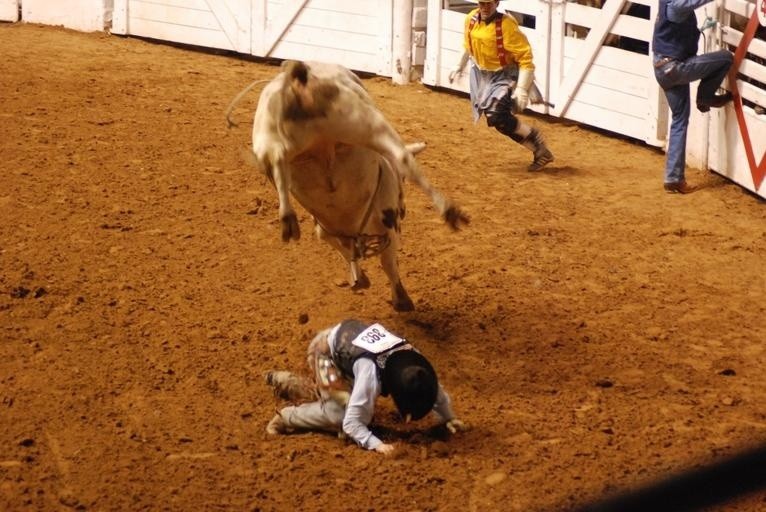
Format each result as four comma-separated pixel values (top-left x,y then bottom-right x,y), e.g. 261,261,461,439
654,56,673,67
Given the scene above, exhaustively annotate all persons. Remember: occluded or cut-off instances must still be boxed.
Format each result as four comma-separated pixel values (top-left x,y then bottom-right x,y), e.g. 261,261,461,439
652,0,734,195
266,319,471,457
448,0,555,171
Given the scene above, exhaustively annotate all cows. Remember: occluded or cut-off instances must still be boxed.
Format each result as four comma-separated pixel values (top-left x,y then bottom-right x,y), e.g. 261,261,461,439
223,58,471,313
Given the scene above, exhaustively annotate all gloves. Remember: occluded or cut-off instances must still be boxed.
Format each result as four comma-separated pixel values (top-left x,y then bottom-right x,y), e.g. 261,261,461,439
511,68,534,112
449,49,469,83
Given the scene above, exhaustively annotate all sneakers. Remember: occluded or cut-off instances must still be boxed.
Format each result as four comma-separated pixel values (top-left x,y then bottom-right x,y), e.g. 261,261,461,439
528,150,554,171
266,409,285,435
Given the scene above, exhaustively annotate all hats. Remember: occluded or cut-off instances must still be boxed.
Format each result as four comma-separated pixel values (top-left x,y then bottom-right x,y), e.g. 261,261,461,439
385,349,437,420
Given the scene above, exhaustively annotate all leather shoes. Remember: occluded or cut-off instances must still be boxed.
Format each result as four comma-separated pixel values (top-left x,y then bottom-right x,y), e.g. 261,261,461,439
664,181,698,194
698,93,732,112
